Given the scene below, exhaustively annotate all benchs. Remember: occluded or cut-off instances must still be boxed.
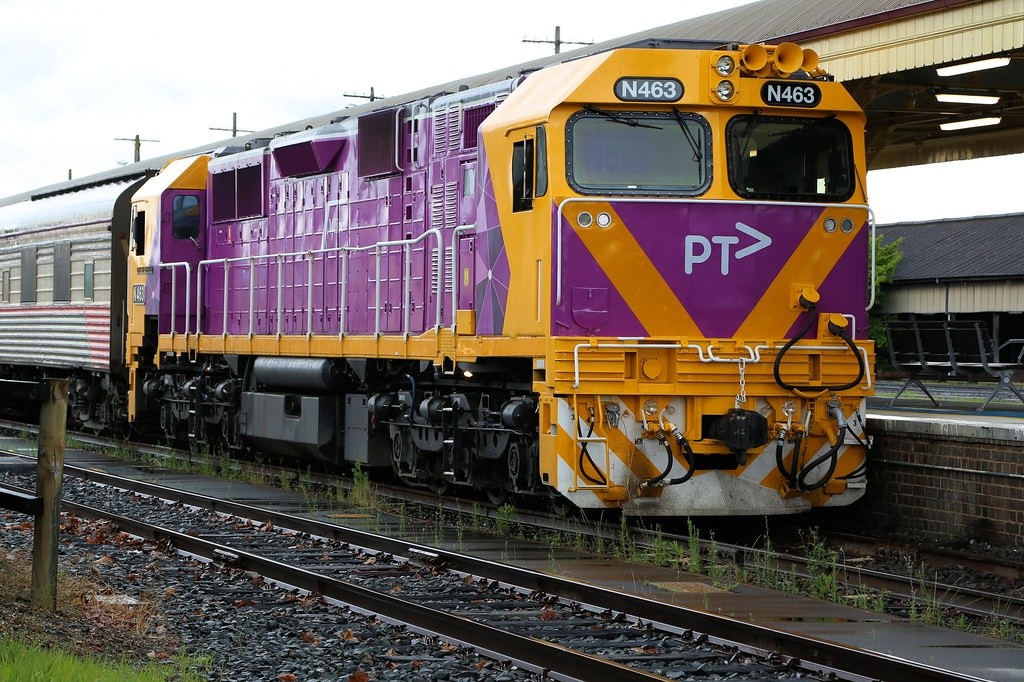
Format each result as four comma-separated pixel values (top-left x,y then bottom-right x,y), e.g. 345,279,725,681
883,317,1024,416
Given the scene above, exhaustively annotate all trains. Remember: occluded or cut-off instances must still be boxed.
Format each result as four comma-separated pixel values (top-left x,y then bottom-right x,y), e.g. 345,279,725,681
0,40,874,532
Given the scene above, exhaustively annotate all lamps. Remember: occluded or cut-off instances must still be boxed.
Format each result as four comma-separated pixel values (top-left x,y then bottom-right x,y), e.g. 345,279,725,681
939,115,1002,131
934,90,1002,105
936,57,1011,77
